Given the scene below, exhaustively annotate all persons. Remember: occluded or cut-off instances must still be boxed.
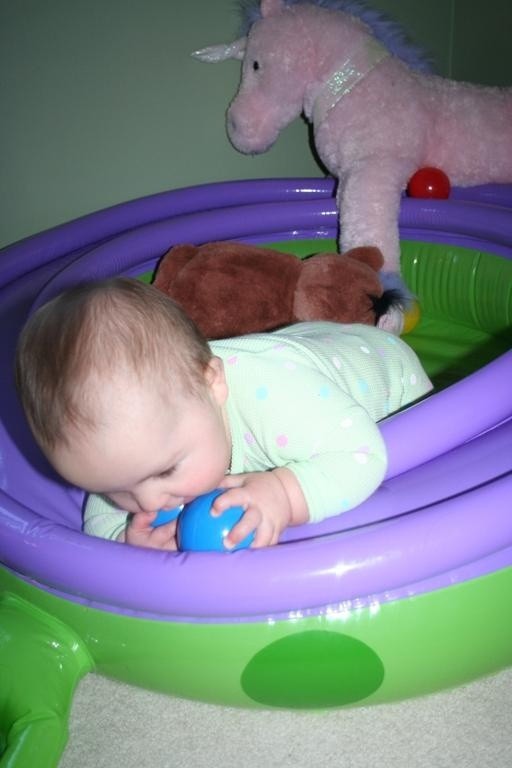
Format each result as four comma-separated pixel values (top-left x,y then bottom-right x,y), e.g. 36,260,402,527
11,274,437,554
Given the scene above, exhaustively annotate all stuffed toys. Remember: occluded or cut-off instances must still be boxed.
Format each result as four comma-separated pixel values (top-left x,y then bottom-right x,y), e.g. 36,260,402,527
185,1,512,335
151,237,389,342
